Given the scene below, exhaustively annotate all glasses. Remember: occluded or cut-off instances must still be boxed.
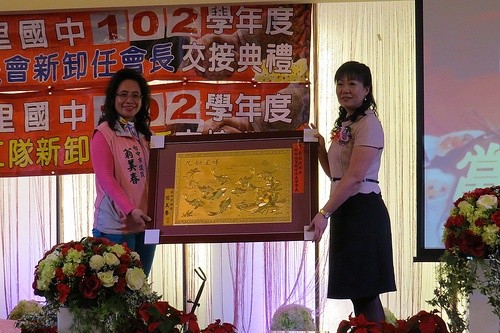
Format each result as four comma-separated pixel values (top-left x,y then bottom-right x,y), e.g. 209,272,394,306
116,92,142,101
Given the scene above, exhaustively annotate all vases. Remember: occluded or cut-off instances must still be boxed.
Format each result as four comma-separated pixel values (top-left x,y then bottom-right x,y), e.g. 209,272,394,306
465,259,500,333
57,307,102,333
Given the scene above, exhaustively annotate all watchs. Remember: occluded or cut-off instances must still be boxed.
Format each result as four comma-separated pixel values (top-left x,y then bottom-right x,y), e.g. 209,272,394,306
320,209,329,219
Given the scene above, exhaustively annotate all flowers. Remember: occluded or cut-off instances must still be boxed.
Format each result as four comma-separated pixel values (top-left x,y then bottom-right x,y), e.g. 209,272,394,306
337,126,351,145
425,185,500,333
336,309,449,333
279,308,314,331
7,236,239,333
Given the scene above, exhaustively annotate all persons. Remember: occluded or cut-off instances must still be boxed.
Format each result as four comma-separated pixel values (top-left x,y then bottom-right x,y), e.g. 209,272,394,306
90,68,156,278
304,61,397,322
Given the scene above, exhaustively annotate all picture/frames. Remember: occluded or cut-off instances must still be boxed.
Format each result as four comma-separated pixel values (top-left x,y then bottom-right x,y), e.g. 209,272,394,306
146,130,319,244
